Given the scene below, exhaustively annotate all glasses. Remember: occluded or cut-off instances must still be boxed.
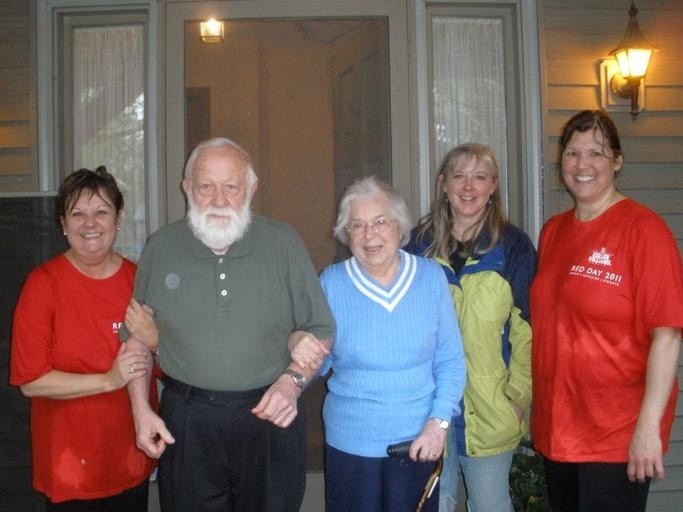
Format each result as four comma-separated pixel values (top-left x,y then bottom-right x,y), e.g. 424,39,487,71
346,218,392,235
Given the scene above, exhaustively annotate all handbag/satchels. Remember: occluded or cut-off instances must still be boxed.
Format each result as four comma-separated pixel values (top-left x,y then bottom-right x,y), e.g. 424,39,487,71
508,438,552,511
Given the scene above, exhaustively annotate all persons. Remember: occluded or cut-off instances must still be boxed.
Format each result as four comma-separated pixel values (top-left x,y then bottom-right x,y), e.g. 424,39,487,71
400,142,538,512
118,136,337,512
528,110,683,512
9,165,159,512
250,175,467,512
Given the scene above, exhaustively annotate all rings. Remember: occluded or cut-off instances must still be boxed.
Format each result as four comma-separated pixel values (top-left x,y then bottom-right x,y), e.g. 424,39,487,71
130,366,134,373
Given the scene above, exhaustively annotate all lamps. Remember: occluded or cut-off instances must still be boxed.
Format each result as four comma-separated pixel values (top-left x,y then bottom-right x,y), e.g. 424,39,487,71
199,21,225,44
609,2,659,123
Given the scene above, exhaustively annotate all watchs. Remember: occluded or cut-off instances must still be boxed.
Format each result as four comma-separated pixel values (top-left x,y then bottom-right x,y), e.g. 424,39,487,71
281,369,306,393
427,417,450,432
